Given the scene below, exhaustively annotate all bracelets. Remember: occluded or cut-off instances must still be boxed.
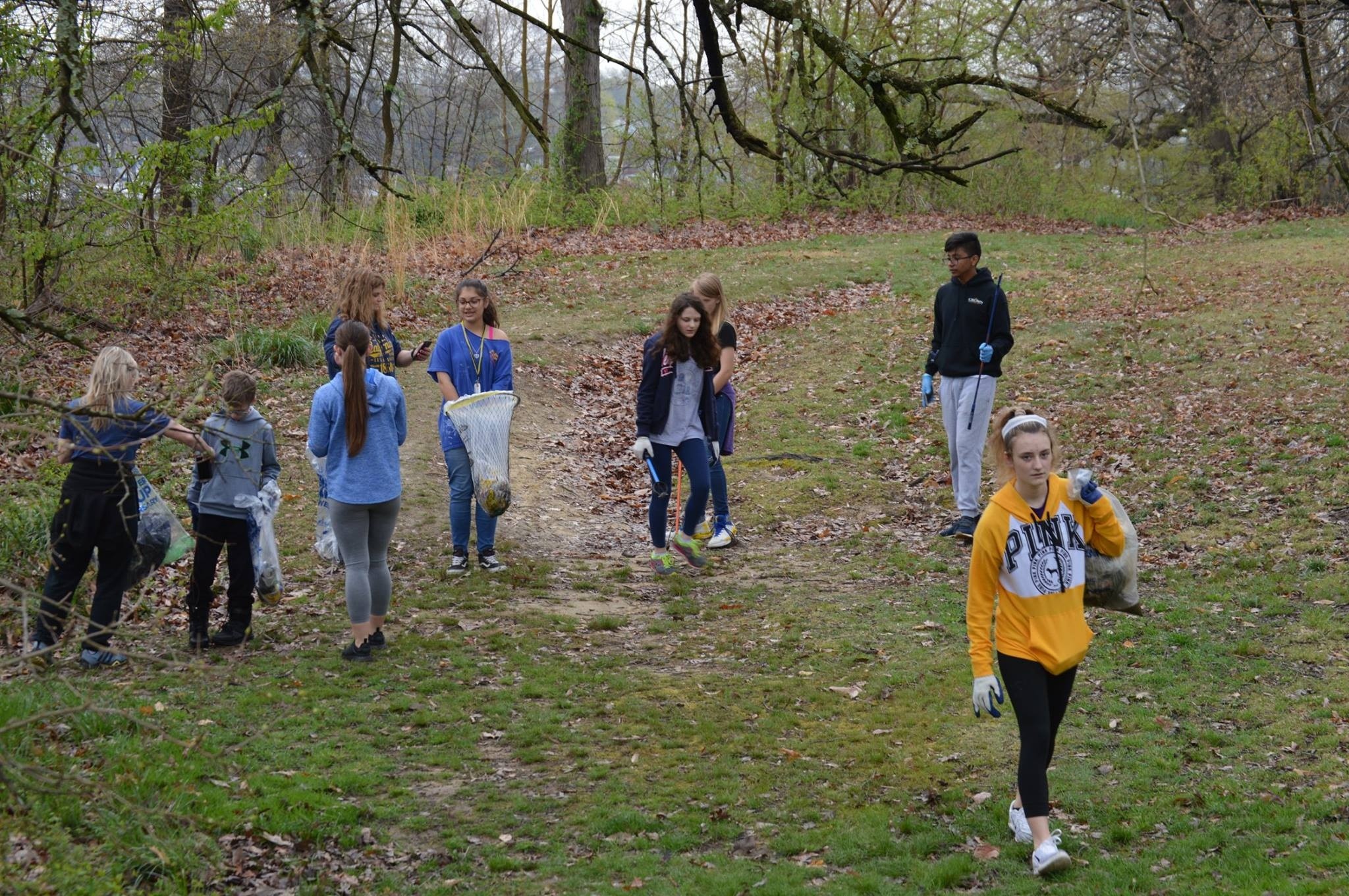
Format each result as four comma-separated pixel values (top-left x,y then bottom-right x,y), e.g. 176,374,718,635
411,348,416,360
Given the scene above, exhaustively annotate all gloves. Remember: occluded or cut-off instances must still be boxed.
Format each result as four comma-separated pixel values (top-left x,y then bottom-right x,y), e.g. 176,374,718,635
1080,480,1102,505
633,436,655,463
972,675,1004,718
708,441,719,468
979,342,993,364
922,374,933,408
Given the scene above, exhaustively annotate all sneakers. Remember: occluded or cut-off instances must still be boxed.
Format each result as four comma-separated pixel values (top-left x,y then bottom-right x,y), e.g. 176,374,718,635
30,640,54,667
665,520,714,541
1007,800,1033,844
341,638,373,661
189,622,209,649
478,548,507,573
939,515,979,538
369,627,386,650
650,550,678,576
446,548,469,575
79,647,127,668
212,619,254,645
1032,829,1072,876
707,515,737,549
672,531,709,570
955,516,976,538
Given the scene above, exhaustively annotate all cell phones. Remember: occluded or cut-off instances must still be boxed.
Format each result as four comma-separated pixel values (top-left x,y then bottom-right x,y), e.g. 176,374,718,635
196,454,212,483
413,341,432,360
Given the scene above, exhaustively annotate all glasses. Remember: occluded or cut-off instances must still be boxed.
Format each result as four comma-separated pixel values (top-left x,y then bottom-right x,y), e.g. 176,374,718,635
942,256,972,266
223,404,250,416
456,298,485,308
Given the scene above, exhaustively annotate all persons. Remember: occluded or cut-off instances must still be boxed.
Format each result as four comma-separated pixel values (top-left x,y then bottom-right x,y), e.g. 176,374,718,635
186,370,281,651
426,279,513,574
324,267,431,562
965,405,1124,877
680,272,738,550
921,233,1015,538
24,346,216,672
309,319,407,662
632,293,720,577
